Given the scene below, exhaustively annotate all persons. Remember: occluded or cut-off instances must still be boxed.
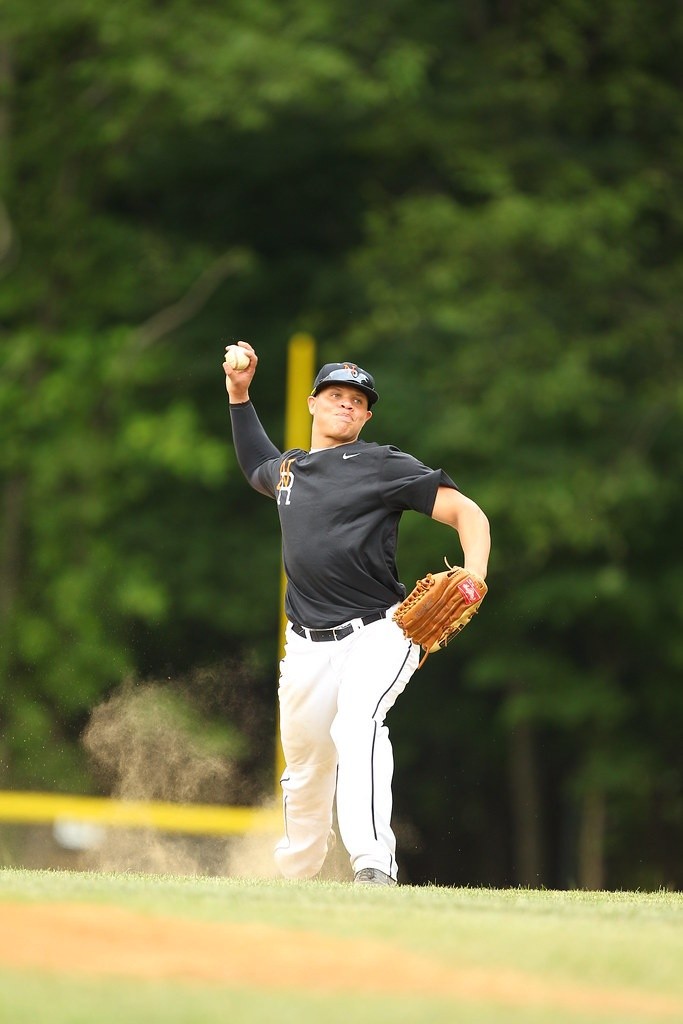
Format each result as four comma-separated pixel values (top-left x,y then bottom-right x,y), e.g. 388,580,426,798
223,341,490,886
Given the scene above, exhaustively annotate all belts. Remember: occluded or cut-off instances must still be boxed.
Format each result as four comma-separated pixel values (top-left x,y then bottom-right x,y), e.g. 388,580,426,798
291,611,387,642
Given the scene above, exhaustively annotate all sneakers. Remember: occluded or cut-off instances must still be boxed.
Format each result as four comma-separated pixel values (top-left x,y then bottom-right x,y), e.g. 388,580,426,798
285,827,336,892
352,868,399,891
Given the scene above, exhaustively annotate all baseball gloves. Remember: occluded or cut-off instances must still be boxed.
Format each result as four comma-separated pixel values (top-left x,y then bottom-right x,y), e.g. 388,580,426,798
390,553,490,671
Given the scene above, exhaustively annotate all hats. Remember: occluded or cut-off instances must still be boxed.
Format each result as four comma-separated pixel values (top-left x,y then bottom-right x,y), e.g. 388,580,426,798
310,362,379,410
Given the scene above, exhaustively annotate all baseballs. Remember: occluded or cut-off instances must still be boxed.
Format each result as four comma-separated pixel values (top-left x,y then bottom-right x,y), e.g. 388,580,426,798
224,345,252,371
